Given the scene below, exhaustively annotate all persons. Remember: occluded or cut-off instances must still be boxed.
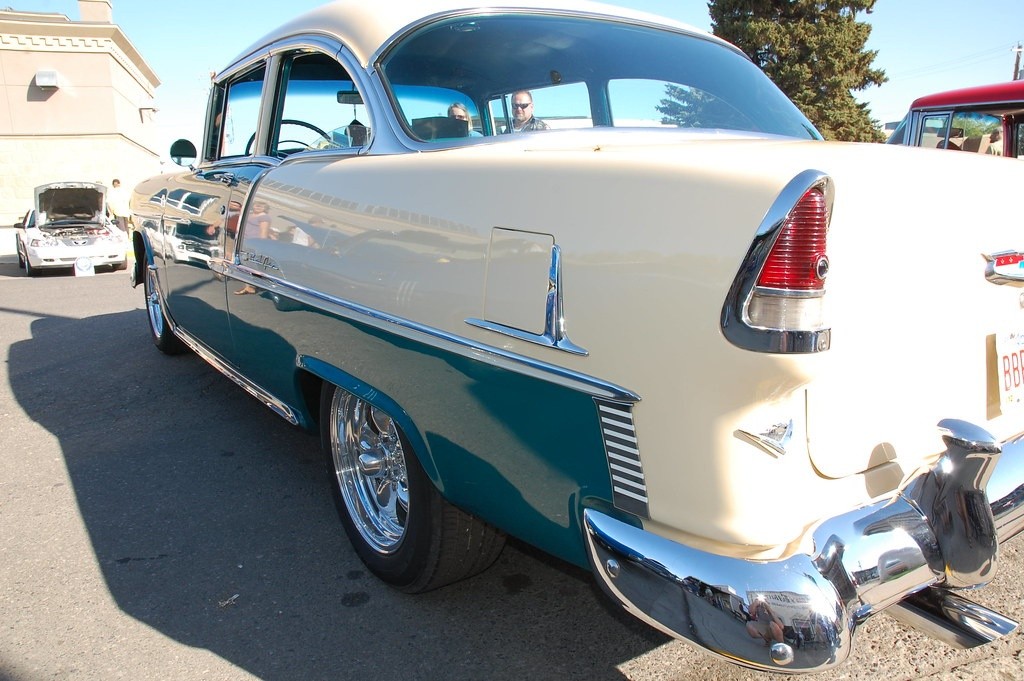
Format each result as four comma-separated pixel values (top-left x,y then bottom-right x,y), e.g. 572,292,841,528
109,180,131,236
505,91,549,132
447,103,482,137
207,201,308,294
746,599,785,645
985,127,1003,156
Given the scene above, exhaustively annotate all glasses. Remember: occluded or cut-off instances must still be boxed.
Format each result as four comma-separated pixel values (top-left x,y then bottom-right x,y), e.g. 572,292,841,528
451,114,466,120
511,103,531,110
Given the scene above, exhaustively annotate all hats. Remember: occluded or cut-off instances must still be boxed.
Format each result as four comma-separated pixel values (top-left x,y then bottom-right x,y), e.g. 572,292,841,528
991,127,1003,138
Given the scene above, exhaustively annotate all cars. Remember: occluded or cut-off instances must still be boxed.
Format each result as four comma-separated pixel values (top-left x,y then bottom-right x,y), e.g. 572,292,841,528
883,79,1024,161
142,218,224,268
13,181,128,277
128,0,1024,674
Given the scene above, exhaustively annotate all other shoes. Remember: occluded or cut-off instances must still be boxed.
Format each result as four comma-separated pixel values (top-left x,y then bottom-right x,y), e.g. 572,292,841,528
234,287,255,295
